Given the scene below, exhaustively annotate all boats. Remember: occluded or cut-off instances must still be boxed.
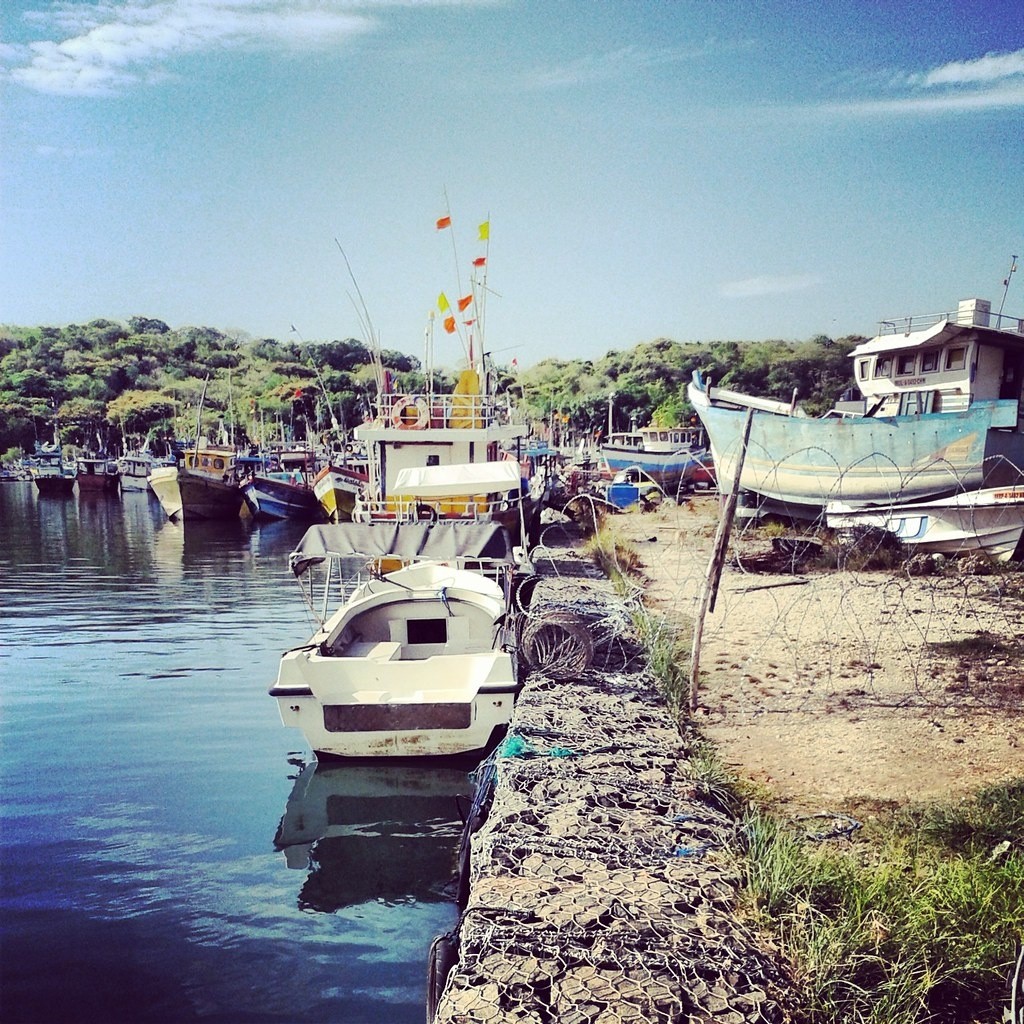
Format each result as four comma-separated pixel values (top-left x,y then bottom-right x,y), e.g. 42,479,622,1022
24,387,549,564
688,251,1023,523
822,476,1024,565
271,560,521,765
268,756,481,870
596,422,717,491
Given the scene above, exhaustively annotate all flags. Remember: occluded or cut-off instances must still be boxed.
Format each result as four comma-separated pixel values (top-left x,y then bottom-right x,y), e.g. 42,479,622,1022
477,221,490,240
462,319,476,326
443,317,455,333
437,294,450,314
457,295,473,312
472,257,487,267
512,359,516,366
437,216,451,228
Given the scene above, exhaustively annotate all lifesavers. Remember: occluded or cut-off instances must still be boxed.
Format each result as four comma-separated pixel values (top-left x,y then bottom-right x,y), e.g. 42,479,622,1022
392,397,430,433
